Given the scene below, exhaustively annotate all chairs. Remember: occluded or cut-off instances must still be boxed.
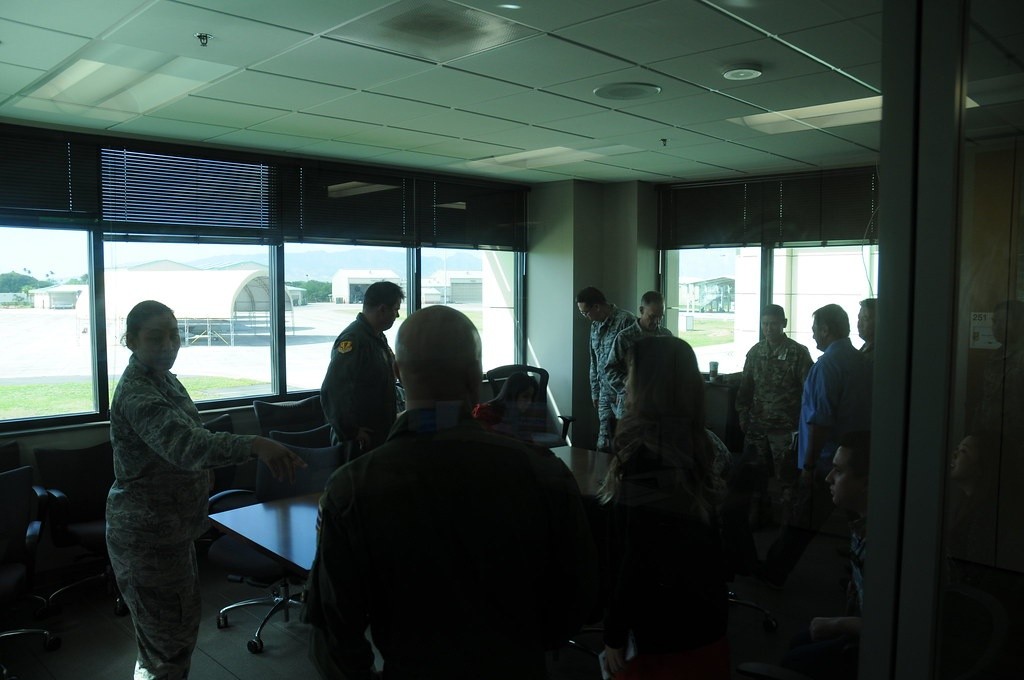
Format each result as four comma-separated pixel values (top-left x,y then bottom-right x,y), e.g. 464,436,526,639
0,393,350,680
486,364,575,450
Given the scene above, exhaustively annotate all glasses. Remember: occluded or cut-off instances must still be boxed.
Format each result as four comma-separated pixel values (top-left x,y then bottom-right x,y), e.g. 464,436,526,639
580,303,597,318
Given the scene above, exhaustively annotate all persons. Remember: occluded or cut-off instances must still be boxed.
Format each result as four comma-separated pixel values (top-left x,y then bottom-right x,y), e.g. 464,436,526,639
105,301,308,680
575,287,638,453
596,336,759,680
472,372,538,443
979,300,1024,462
301,305,599,680
734,305,814,511
604,291,671,419
760,306,874,589
858,299,878,368
943,430,1020,564
780,430,870,680
320,282,404,464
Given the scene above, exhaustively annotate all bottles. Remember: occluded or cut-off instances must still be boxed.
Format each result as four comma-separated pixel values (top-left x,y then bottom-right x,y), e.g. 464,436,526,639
710,362,718,383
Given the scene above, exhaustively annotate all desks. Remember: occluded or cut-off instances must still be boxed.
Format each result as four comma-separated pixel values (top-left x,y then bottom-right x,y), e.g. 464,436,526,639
208,448,614,653
